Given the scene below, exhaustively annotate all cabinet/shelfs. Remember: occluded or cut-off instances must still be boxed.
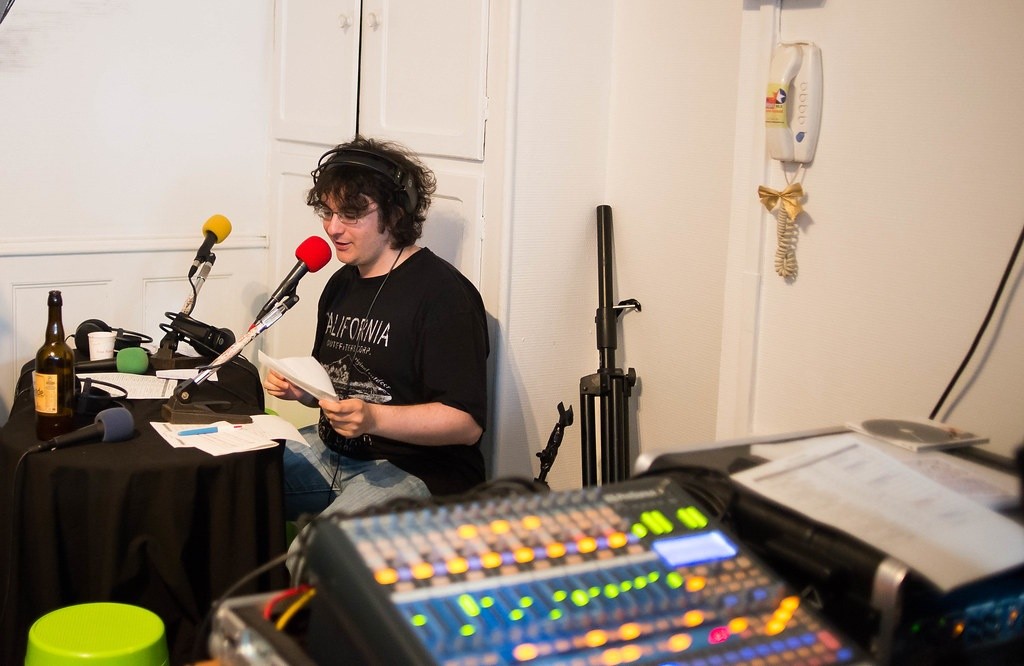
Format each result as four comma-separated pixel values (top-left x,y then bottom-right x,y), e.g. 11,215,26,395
262,0,743,497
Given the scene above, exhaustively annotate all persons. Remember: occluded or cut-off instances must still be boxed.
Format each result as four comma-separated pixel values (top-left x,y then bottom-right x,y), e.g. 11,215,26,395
260,131,491,584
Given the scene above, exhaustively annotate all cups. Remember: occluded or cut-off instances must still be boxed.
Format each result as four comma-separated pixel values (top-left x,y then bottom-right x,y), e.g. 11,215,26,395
87,331,117,362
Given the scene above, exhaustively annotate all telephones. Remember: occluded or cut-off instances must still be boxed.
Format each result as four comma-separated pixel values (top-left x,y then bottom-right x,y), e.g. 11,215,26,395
764,41,825,164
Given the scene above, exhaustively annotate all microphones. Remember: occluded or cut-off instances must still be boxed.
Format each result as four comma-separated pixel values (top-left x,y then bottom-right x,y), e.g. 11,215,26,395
187,214,233,276
249,237,323,330
29,406,134,456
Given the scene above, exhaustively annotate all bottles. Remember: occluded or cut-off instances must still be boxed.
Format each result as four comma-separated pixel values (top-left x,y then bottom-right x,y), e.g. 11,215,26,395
33,290,74,440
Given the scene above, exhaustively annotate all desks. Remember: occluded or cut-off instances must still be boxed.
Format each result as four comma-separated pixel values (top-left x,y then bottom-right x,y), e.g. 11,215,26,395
1,348,288,491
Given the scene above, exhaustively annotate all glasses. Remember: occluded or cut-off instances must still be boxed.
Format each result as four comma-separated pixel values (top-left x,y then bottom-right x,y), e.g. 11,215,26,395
315,204,380,223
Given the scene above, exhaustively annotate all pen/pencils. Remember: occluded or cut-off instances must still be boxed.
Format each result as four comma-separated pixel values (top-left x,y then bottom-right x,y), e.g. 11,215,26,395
178,423,243,436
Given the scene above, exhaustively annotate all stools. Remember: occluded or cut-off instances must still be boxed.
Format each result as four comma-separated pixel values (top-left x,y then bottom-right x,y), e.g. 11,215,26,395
24,602,172,666
